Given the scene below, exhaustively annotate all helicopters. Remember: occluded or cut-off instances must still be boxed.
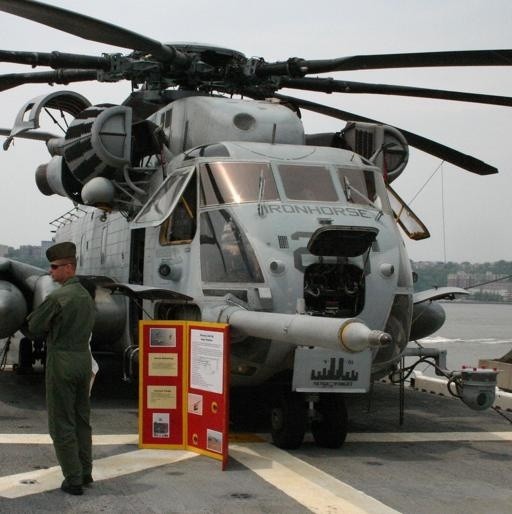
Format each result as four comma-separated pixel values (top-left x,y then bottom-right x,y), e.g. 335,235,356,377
0,0,512,454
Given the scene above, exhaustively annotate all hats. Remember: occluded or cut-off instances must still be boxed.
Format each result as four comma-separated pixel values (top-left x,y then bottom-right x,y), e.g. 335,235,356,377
46,242,76,262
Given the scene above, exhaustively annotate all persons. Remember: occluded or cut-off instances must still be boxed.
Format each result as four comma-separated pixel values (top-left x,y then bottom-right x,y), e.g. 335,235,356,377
26,242,97,496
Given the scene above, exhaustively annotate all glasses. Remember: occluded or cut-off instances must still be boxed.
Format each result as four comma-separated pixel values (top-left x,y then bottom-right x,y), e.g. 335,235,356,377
50,263,74,270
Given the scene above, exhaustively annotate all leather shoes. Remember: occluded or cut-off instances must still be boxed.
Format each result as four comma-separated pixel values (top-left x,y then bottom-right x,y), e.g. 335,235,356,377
61,475,93,495
